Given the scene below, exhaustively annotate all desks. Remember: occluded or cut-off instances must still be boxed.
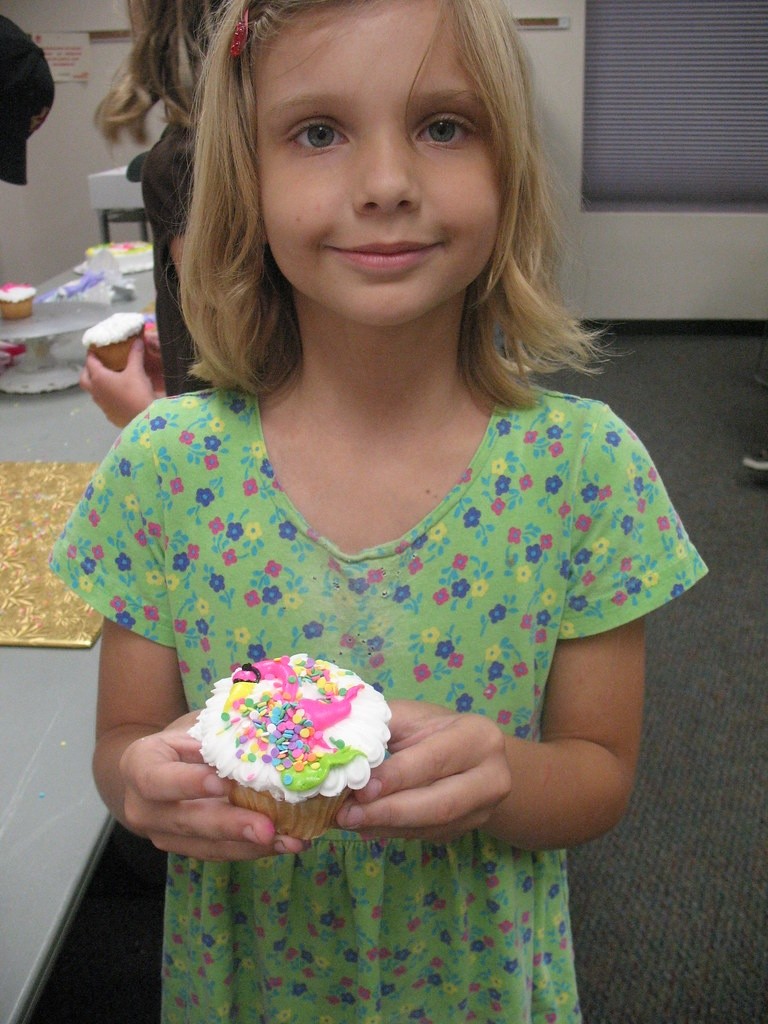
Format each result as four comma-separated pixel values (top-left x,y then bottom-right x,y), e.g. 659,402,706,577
0,268,174,1023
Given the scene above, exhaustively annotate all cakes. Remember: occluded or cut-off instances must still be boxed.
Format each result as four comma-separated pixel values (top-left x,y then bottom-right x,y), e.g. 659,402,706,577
85,240,156,272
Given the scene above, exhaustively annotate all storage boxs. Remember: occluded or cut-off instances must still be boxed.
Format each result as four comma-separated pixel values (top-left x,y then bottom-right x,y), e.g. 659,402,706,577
88,165,145,211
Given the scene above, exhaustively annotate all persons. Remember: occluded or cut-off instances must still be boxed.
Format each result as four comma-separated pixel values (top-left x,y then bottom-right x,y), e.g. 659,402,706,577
50,0,710,1024
0,14,54,369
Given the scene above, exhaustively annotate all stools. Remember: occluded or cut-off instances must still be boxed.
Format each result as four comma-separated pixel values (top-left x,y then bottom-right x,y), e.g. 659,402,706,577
99,209,152,246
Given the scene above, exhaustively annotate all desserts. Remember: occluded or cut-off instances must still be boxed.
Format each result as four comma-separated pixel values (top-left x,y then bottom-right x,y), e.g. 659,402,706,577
187,653,392,840
81,312,149,372
0,282,37,319
